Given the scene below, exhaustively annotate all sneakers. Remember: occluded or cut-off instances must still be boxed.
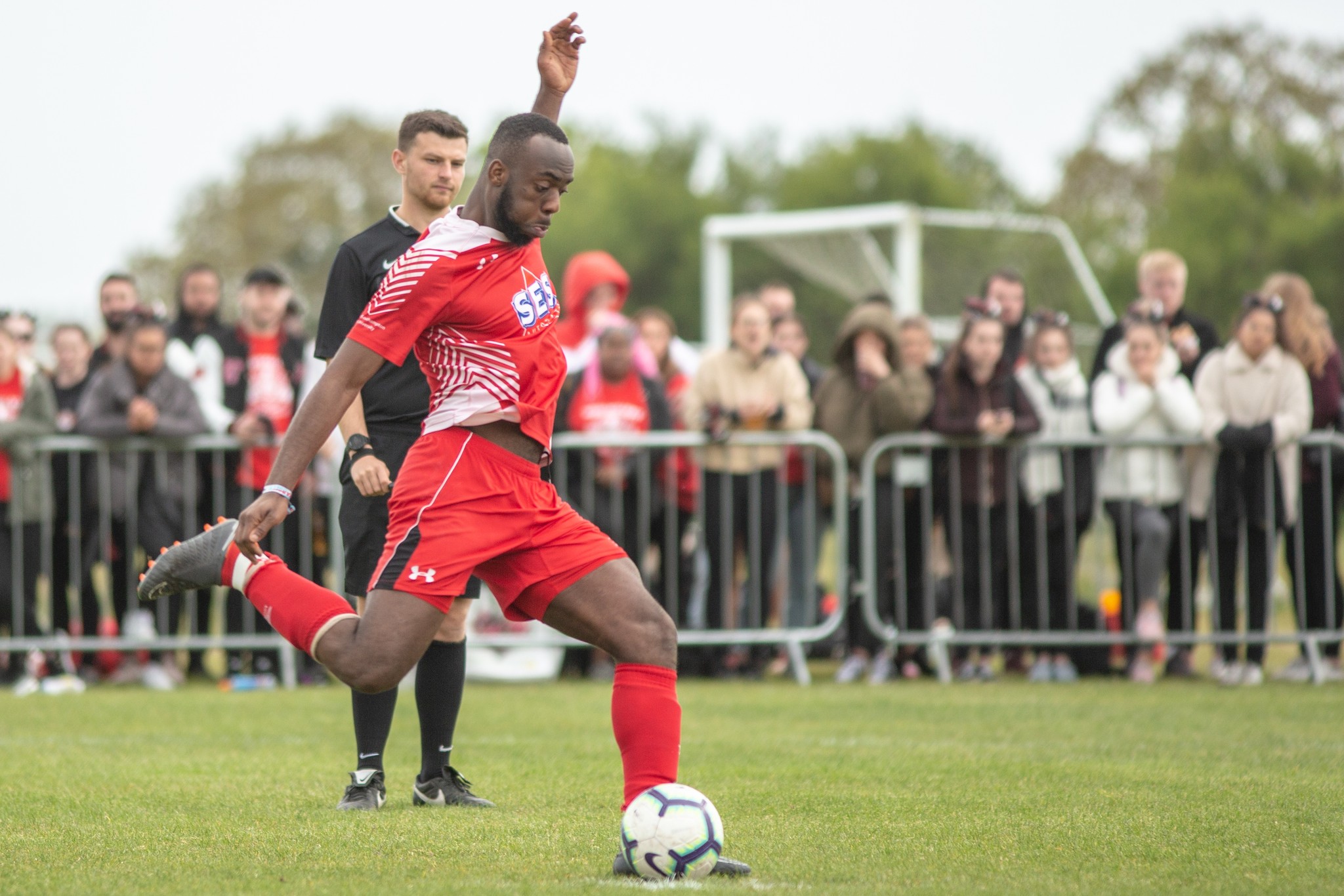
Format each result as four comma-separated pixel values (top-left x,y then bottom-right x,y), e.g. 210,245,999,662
339,768,387,810
136,515,239,606
613,850,751,876
411,766,495,808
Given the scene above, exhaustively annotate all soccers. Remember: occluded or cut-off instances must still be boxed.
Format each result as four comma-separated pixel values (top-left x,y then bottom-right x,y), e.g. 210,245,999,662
618,784,724,880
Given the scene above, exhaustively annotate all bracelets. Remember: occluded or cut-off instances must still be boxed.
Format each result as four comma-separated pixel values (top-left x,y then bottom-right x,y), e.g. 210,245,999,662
262,484,295,514
350,448,376,463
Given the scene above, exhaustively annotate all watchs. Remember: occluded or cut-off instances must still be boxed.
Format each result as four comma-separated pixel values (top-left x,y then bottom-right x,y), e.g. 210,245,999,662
346,433,372,451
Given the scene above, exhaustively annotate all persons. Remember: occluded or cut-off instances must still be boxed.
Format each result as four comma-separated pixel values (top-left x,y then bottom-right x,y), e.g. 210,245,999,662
0,246,1343,685
138,12,752,880
314,107,493,810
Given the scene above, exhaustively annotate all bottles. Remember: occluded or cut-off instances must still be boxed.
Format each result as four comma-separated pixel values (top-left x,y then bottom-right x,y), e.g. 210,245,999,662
218,672,276,692
